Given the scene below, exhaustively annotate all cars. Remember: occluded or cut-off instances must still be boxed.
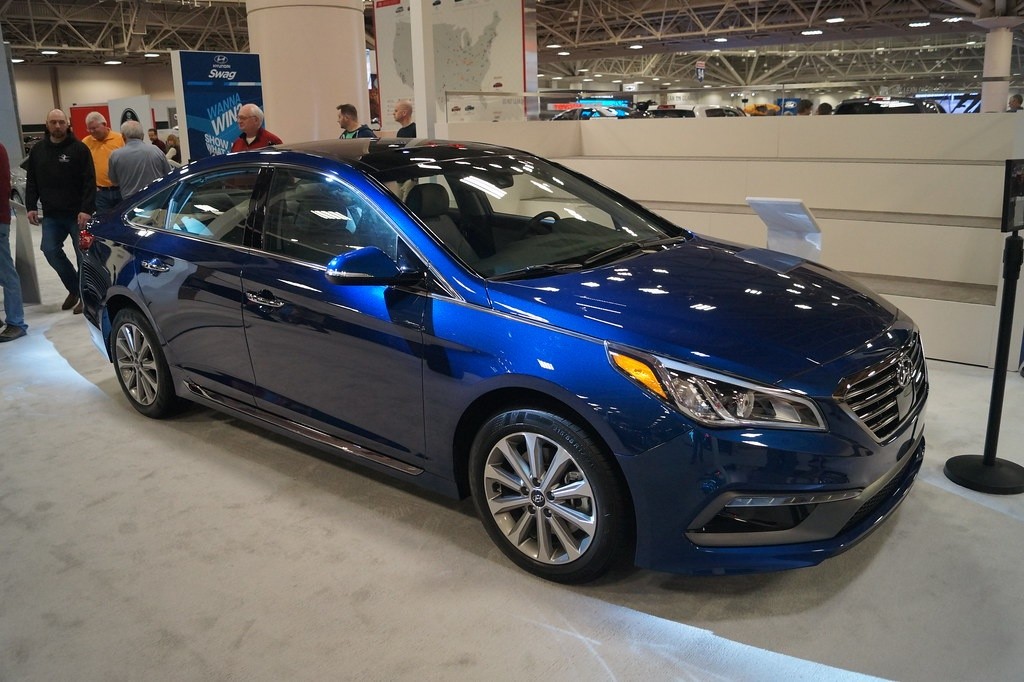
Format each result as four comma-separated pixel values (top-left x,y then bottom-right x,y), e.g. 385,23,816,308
646,104,748,118
834,96,949,114
78,136,930,588
549,108,654,120
9,153,43,218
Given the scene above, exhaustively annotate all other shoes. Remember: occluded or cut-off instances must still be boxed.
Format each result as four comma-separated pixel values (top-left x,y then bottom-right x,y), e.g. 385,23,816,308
73,301,83,314
62,293,78,311
0,324,27,342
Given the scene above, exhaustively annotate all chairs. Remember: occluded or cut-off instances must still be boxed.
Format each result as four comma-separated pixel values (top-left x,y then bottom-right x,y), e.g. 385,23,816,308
283,198,361,268
405,183,481,267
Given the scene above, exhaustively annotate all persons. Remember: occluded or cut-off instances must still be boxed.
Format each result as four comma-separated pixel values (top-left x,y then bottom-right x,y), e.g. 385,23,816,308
83,111,126,214
1007,94,1024,112
25,109,96,315
393,100,418,202
818,103,832,115
0,143,28,343
231,104,294,192
166,134,181,163
148,129,167,155
797,99,813,116
337,104,376,139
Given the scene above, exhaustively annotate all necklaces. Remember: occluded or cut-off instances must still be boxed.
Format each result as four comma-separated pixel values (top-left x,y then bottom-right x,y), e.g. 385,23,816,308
108,121,173,212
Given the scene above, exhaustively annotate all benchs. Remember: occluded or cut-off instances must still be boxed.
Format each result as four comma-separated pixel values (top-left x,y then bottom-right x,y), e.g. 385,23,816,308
174,182,326,245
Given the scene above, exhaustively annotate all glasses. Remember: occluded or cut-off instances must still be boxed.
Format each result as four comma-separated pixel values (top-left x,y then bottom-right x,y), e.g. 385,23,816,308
87,129,98,133
236,115,256,120
